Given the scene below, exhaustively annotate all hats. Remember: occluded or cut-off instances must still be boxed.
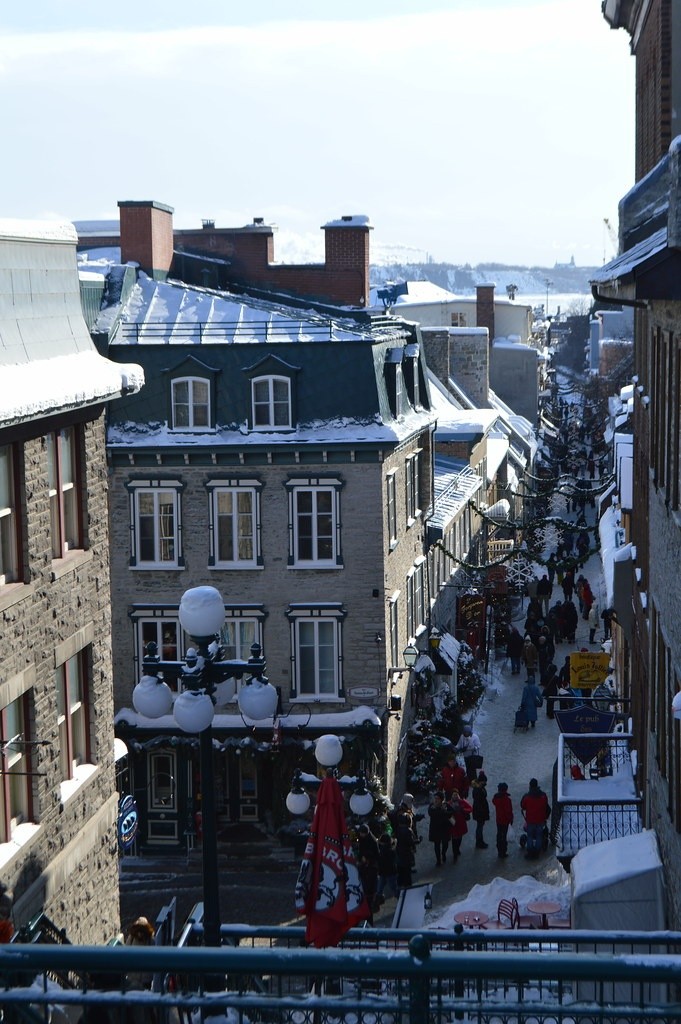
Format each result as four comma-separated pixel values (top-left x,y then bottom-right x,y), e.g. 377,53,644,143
529,778,538,788
524,635,531,641
478,771,487,782
498,783,508,791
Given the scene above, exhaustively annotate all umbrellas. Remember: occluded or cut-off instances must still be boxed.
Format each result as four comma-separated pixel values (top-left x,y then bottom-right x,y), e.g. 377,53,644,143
295,767,371,995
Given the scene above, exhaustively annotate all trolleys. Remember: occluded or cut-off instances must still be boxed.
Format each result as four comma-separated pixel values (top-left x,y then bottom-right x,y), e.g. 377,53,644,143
513,705,528,733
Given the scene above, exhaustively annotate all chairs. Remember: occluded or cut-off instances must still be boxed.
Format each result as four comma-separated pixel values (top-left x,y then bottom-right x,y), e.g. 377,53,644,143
478,899,514,951
512,897,542,946
547,904,571,929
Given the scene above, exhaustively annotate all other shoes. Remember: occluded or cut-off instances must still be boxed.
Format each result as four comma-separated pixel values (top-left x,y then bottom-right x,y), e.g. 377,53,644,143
435,860,441,866
458,849,461,855
498,852,508,857
476,841,488,849
454,853,457,860
589,641,596,644
525,851,539,860
442,854,446,862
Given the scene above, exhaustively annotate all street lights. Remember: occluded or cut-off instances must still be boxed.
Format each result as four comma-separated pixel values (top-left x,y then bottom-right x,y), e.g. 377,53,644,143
284,733,374,995
131,588,281,1023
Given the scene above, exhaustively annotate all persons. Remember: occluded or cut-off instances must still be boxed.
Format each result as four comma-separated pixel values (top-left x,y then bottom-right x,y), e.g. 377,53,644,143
471,770,490,849
506,626,555,684
395,792,425,887
521,676,543,730
559,397,606,519
524,600,578,645
492,783,514,858
561,571,596,625
358,823,399,907
526,575,553,617
520,778,548,861
589,602,600,645
437,753,469,806
545,505,590,586
427,789,473,866
543,647,593,720
601,601,614,639
125,916,155,990
456,725,481,787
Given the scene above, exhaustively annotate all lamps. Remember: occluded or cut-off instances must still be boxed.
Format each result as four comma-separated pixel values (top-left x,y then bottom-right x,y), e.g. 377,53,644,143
429,627,442,651
403,643,419,673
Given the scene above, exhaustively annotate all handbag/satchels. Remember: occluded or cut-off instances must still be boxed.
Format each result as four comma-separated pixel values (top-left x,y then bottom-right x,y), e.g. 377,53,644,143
542,688,548,699
469,745,483,769
535,699,541,707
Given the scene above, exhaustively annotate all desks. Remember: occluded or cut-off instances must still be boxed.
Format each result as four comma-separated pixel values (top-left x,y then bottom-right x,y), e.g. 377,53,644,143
454,911,489,929
528,902,562,930
